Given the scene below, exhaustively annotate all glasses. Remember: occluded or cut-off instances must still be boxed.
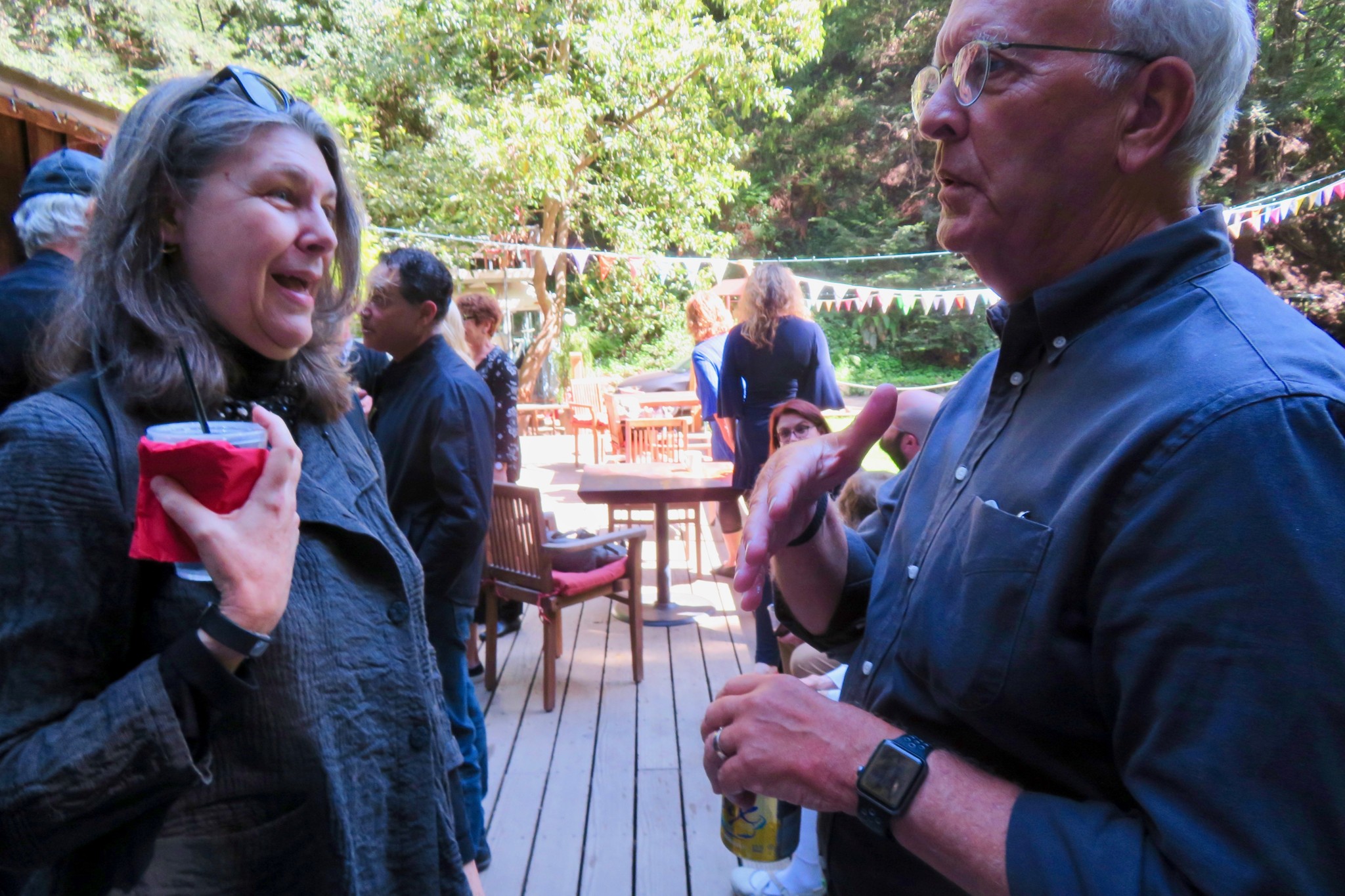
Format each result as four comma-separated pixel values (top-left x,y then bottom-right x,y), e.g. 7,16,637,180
773,425,815,442
910,38,1156,124
189,65,295,115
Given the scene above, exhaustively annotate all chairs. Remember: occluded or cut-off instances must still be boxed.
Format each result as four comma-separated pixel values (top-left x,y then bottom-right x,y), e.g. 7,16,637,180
480,378,702,714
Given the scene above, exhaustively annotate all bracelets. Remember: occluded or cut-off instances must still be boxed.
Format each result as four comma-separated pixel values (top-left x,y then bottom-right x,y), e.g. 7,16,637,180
785,490,832,547
196,602,271,659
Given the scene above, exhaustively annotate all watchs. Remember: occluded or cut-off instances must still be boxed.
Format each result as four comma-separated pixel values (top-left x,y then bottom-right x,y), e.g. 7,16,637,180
854,732,941,836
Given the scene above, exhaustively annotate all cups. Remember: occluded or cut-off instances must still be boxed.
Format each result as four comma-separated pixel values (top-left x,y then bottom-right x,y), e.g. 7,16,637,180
146,421,268,580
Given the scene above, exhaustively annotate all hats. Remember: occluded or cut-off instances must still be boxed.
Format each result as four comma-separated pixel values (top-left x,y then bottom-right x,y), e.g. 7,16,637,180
19,149,108,202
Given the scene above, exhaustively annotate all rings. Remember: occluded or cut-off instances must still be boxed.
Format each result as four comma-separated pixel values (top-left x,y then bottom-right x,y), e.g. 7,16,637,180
712,726,728,762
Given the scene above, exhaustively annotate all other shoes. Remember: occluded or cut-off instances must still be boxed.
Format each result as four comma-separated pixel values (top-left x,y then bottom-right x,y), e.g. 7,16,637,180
709,564,735,578
468,662,486,684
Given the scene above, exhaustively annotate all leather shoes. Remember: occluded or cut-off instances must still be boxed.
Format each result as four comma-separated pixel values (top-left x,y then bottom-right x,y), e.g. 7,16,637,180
479,616,521,641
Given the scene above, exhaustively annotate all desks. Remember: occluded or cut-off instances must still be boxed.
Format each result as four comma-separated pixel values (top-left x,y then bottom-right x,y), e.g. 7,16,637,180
578,460,742,625
616,391,700,408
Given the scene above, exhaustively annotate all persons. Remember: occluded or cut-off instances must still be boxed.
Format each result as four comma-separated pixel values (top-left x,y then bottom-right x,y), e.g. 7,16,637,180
452,294,528,640
684,293,765,575
0,63,486,896
0,150,111,400
351,246,492,871
709,0,1345,895
713,260,847,506
423,301,488,681
323,305,393,431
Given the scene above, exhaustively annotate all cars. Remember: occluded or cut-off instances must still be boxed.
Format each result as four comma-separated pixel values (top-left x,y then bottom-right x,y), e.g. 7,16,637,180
617,357,692,417
